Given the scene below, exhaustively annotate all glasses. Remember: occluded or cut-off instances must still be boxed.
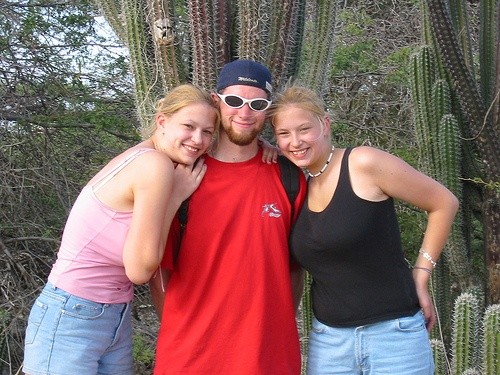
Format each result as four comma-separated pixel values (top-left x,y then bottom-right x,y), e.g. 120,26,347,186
215,92,273,112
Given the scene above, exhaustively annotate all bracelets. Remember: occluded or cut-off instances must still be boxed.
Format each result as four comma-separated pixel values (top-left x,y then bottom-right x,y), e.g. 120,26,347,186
412,266,432,275
418,247,437,268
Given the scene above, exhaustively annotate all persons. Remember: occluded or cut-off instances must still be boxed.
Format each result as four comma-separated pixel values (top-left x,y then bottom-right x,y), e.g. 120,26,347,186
22,84,280,375
267,83,459,375
148,59,308,375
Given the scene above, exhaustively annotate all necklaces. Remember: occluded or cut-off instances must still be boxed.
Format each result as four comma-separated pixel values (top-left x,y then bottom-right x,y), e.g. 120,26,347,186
305,145,335,177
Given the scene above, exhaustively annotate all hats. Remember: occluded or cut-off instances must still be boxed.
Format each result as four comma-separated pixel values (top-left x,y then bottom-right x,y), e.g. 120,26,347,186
216,59,272,97
